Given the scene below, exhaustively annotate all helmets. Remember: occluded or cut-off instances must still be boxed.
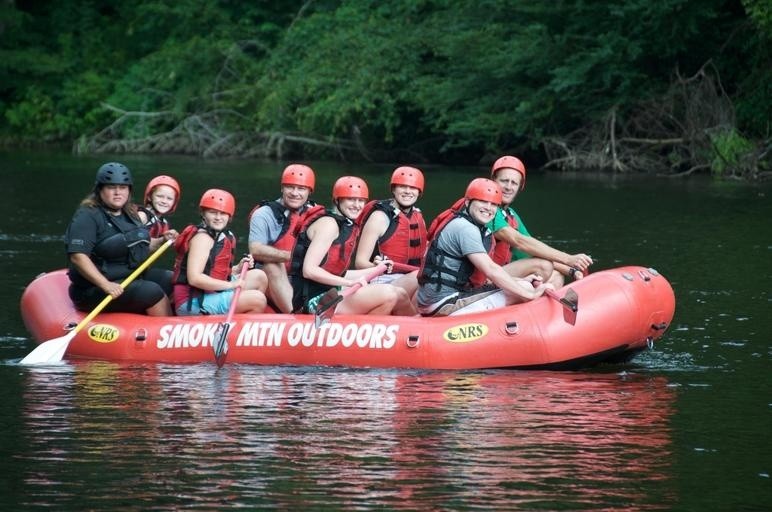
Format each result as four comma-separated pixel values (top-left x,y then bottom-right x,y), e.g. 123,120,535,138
330,175,370,204
389,164,425,201
198,186,236,224
465,177,502,209
143,175,181,213
92,160,135,194
279,164,316,196
491,155,527,193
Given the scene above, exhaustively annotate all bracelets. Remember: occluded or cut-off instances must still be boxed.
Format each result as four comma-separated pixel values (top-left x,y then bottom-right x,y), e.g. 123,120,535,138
568,267,579,278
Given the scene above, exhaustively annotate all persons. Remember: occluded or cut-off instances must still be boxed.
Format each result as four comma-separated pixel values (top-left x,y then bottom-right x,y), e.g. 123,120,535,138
247,164,324,313
347,164,428,307
136,174,179,274
64,163,180,320
413,176,566,318
171,187,271,316
483,155,593,293
287,174,418,319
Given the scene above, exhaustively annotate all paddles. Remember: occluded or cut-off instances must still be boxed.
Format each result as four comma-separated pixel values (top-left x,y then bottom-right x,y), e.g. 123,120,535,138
213,253,250,367
307,256,391,330
18,234,177,366
533,280,578,325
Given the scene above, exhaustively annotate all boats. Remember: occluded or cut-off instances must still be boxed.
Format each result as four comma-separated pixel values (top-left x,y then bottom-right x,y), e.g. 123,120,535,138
17,262,675,374
